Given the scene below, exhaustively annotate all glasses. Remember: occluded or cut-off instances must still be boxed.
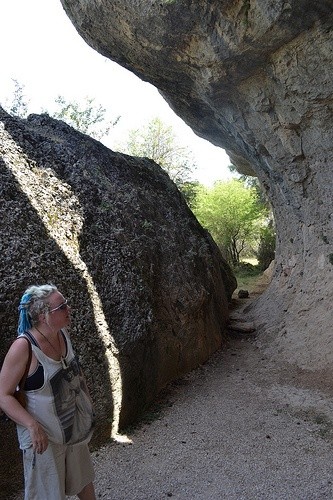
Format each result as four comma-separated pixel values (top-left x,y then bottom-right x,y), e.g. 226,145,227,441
49,299,68,313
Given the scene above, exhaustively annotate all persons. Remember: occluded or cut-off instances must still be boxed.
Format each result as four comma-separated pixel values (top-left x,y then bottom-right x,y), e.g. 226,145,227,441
62,369,94,440
1,284,100,500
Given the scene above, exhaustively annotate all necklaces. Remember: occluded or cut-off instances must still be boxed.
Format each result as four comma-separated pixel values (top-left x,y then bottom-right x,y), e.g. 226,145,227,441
33,325,70,370
